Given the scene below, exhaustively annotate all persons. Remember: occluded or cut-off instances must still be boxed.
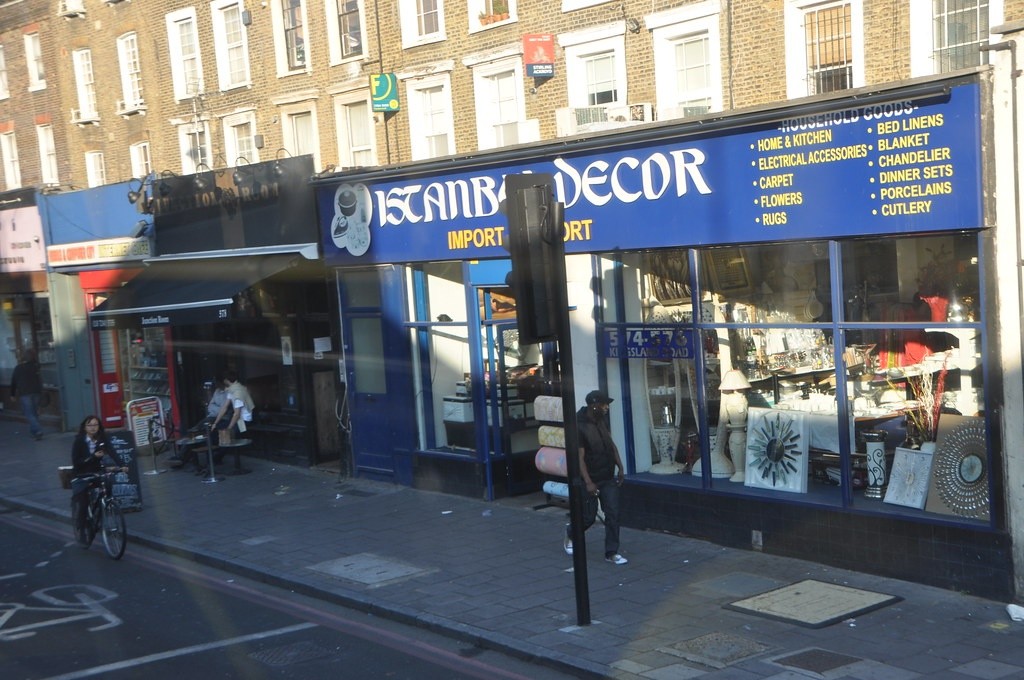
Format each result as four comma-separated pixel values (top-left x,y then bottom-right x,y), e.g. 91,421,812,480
562,390,629,564
72,416,128,529
168,375,234,461
207,370,253,465
10,350,45,440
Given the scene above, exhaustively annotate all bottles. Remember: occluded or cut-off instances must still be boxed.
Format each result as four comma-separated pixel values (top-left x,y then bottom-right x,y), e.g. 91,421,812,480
739,329,831,378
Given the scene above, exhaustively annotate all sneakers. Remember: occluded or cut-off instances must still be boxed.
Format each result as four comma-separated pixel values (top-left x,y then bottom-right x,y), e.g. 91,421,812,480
606,553,627,565
563,530,573,555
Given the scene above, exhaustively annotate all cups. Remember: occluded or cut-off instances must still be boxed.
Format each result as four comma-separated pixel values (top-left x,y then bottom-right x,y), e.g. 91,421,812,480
783,390,868,412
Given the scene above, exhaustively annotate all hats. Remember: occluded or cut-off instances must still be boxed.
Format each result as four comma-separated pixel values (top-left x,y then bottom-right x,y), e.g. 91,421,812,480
585,390,613,405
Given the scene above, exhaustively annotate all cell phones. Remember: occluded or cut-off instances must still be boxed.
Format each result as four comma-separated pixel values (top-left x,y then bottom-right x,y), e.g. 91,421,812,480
98,443,105,451
592,490,600,497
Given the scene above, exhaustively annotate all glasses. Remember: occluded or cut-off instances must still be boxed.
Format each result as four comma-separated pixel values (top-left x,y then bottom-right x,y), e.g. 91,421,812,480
85,424,99,428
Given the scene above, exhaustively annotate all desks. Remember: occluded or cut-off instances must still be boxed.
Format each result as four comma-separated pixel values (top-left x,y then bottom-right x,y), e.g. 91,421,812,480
218,438,254,477
174,435,210,477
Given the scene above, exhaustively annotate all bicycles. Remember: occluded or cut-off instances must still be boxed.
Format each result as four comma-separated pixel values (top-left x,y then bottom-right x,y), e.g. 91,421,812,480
68,469,129,561
